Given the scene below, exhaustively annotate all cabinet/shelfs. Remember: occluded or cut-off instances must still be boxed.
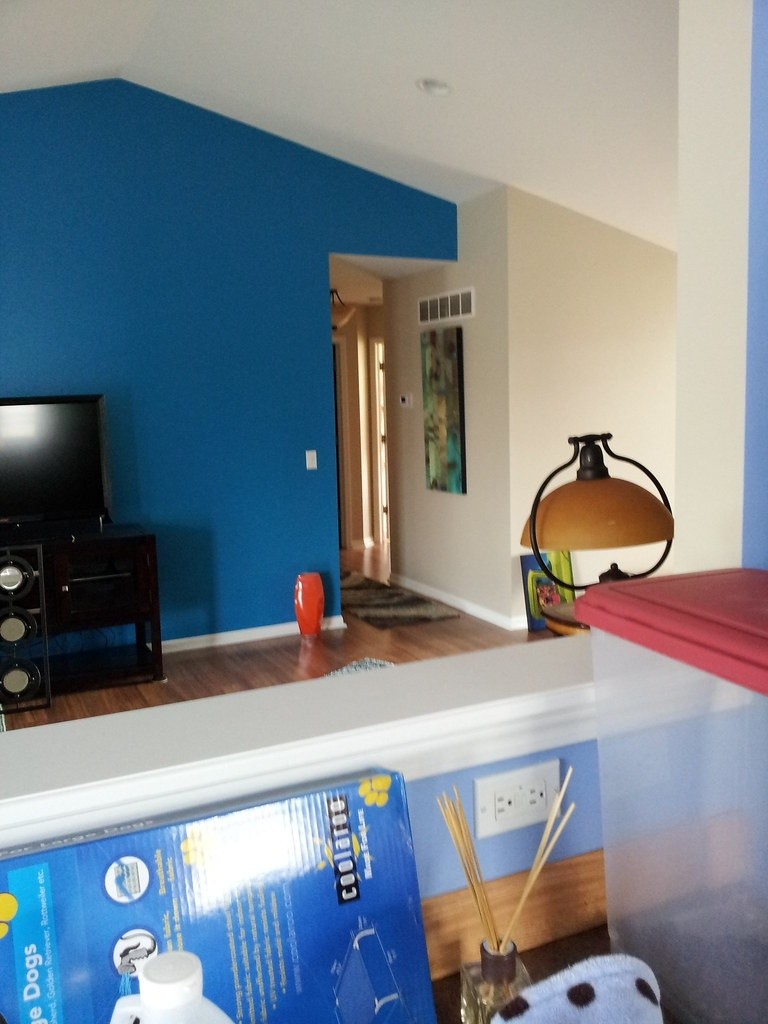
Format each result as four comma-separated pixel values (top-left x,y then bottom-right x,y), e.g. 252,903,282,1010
1,523,162,705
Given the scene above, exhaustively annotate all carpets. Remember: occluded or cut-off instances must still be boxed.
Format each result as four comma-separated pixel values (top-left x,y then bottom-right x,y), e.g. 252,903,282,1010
339,566,460,631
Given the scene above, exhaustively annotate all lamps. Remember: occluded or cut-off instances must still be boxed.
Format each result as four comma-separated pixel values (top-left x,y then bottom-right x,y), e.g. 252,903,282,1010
518,431,672,636
330,288,357,331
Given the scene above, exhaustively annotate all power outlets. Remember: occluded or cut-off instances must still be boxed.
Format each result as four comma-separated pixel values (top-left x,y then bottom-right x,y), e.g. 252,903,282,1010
474,759,561,839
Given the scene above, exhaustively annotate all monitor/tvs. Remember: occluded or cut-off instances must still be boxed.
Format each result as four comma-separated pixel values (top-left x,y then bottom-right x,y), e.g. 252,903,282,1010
0,393,113,545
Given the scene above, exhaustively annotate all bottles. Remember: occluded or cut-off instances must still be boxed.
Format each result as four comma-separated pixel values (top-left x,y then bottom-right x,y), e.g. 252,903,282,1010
460,937,535,1024
108,949,240,1024
